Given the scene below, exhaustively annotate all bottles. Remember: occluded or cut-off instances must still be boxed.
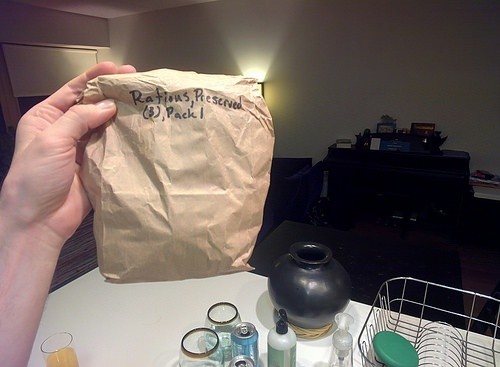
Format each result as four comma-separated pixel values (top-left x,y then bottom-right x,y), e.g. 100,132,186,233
267,243,352,340
328,313,353,367
267,309,297,367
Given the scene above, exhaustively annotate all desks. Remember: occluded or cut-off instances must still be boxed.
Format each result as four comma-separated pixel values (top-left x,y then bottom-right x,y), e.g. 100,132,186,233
24,266,500,367
309,142,471,247
471,184,500,257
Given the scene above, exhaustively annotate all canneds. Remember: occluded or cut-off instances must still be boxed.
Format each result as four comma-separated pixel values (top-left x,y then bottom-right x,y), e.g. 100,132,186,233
229,322,259,367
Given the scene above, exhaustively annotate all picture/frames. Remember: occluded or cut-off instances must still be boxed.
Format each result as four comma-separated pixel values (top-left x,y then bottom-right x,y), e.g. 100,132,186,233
377,123,396,134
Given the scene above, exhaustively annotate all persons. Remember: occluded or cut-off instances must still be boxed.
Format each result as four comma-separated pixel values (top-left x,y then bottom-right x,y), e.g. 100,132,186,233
0,60,138,367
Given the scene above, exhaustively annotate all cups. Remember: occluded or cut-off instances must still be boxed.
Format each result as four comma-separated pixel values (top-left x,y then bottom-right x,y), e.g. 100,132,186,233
204,301,243,367
40,332,80,367
365,330,419,367
178,327,224,367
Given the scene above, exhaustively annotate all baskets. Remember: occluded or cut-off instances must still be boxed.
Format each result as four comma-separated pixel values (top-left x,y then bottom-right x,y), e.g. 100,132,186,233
358,277,500,367
